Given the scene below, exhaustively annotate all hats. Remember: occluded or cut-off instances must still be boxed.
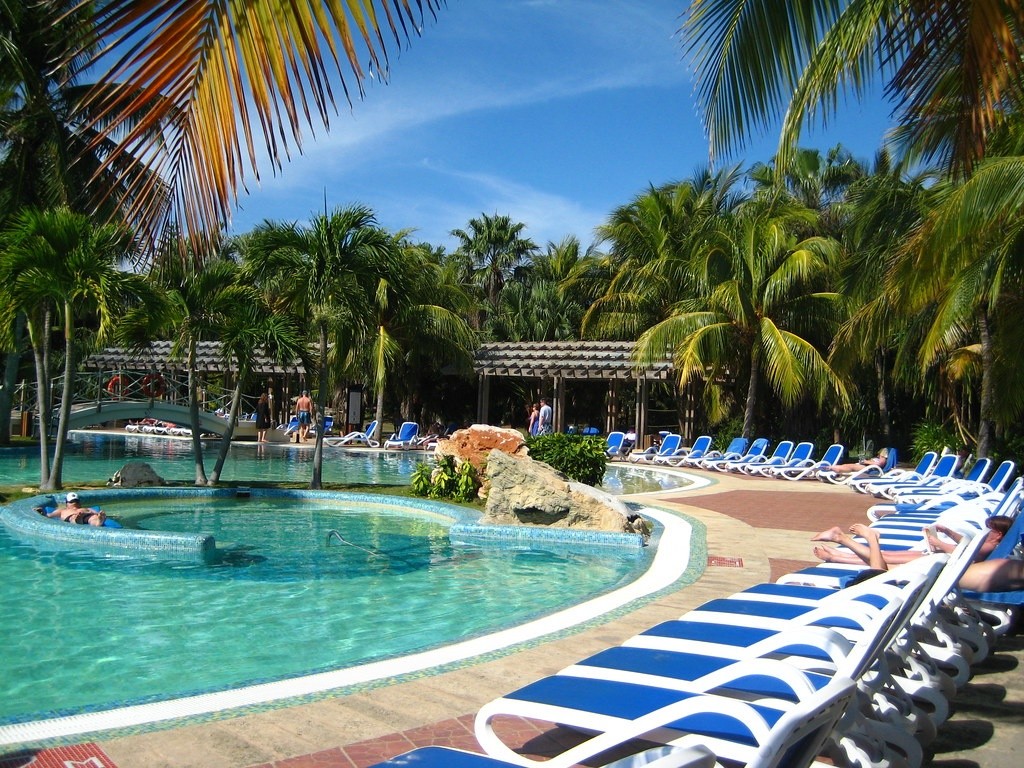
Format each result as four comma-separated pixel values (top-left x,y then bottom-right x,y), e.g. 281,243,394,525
66,492,80,502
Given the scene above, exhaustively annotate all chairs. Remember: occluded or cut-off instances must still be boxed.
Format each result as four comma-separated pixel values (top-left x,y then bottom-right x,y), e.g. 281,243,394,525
125,412,1024,768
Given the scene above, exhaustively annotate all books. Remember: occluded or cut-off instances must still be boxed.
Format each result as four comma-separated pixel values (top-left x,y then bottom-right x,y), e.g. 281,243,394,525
859,454,866,464
922,524,937,552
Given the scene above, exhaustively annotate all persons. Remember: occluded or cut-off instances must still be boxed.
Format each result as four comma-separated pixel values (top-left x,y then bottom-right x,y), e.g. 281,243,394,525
819,448,888,473
622,429,635,447
255,393,271,442
956,445,972,470
538,398,552,435
528,402,540,436
810,516,1024,594
33,492,106,526
296,390,313,443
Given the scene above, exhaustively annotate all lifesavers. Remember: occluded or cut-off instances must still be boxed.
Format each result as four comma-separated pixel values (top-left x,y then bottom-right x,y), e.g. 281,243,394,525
108,376,129,401
142,372,166,397
